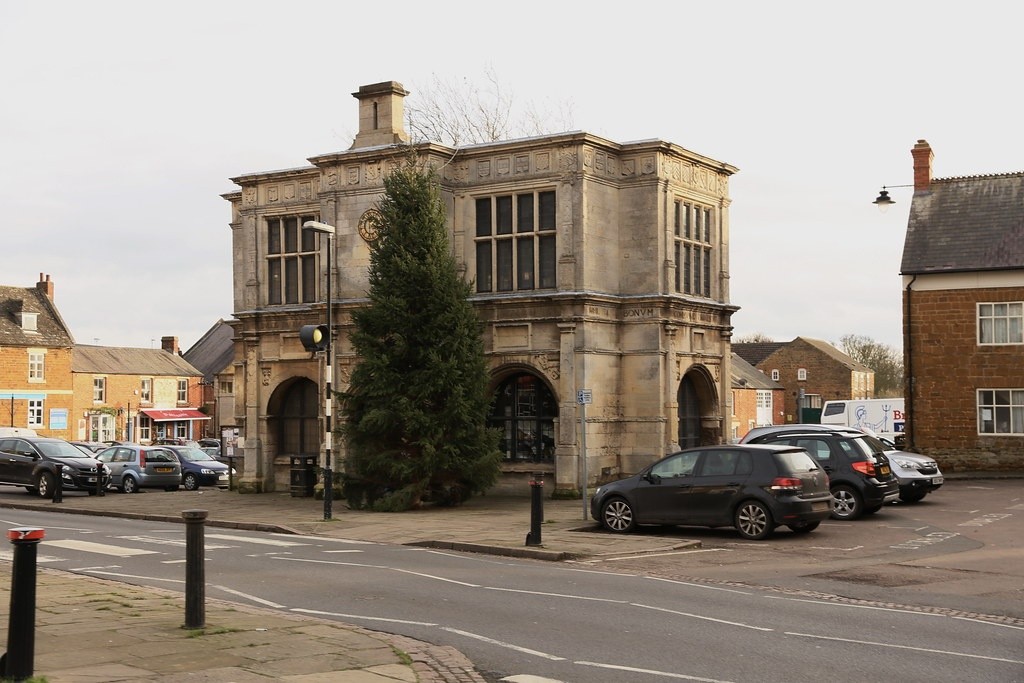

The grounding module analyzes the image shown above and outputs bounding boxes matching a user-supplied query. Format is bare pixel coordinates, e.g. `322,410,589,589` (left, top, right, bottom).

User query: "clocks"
358,208,383,241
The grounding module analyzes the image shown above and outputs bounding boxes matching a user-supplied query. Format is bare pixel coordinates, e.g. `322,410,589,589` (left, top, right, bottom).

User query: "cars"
0,436,113,499
70,438,222,458
158,445,237,491
93,445,183,494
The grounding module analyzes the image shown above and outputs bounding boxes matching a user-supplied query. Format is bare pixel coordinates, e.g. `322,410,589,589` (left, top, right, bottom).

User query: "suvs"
736,423,944,520
591,444,835,540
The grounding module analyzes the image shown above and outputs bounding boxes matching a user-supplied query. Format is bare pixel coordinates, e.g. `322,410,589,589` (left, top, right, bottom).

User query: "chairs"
702,453,722,475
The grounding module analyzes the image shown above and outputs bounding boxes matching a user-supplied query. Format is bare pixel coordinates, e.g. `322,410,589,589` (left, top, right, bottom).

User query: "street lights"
299,220,335,520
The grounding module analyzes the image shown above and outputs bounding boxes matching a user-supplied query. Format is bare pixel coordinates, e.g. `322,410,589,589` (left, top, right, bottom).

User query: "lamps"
873,184,915,213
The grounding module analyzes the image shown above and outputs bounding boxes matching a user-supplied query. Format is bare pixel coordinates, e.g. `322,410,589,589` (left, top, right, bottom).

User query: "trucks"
821,396,905,443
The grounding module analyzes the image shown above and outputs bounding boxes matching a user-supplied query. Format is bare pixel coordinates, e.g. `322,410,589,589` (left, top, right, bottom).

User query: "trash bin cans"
895,436,905,451
290,454,318,497
